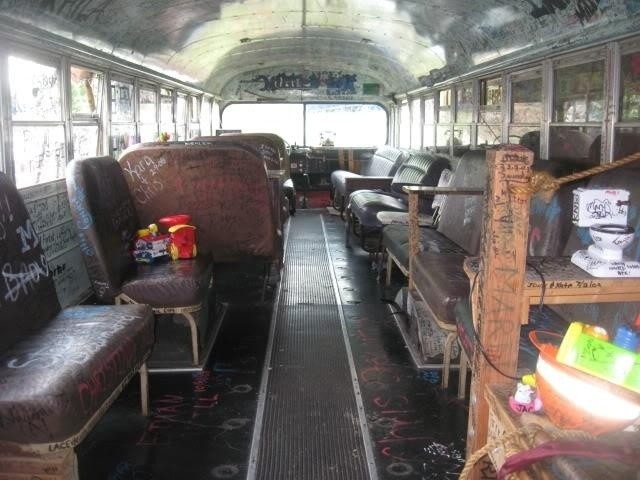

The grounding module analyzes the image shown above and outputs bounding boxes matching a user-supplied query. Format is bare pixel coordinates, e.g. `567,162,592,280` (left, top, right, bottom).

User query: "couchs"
117,142,285,301
342,153,454,252
66,155,216,365
453,165,639,400
1,173,157,476
331,145,402,212
380,150,601,390
